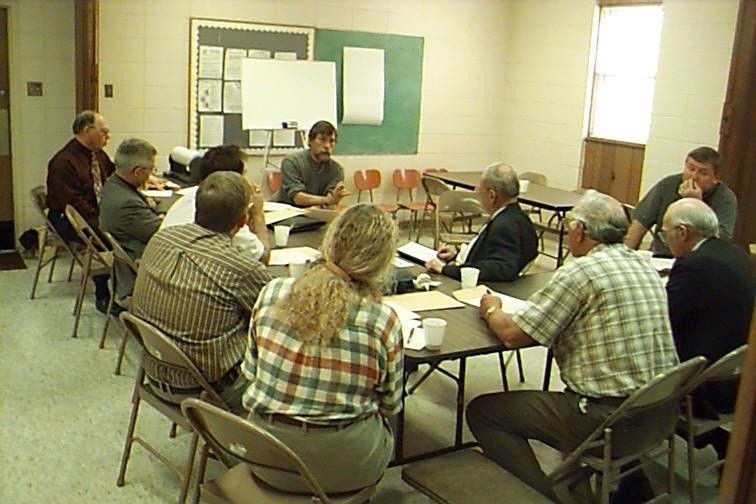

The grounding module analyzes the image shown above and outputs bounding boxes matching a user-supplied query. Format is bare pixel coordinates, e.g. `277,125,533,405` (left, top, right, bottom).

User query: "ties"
92,152,103,206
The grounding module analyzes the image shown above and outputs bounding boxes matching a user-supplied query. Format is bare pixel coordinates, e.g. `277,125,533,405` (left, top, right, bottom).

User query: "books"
397,241,448,271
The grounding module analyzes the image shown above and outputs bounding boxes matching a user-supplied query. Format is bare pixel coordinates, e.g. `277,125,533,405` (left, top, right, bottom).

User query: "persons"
464,189,681,504
656,198,756,487
131,172,273,415
623,146,737,256
97,137,164,302
394,163,539,373
45,111,128,317
241,203,404,494
156,144,271,267
265,120,351,207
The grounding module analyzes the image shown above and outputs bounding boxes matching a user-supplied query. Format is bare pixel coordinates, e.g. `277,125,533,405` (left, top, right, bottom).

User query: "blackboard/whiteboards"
240,57,338,131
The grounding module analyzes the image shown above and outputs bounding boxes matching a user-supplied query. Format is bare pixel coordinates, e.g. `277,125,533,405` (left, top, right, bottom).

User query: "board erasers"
283,122,298,130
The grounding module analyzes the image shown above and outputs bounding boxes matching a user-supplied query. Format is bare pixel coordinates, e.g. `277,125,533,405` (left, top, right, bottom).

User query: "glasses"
657,226,679,243
133,164,158,176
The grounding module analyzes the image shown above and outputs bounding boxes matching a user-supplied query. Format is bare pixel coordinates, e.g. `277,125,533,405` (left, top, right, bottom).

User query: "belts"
145,367,237,396
264,410,353,430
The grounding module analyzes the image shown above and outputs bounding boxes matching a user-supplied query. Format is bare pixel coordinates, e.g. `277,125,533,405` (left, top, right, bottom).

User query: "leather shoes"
97,295,128,316
609,476,655,504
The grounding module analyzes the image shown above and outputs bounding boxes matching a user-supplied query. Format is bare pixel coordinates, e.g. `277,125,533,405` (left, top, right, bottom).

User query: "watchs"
484,305,499,325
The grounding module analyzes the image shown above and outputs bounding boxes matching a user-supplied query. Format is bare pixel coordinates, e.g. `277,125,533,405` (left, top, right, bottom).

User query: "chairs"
622,204,636,224
65,204,113,337
181,397,384,504
518,171,546,251
673,344,748,504
99,226,138,375
30,185,84,298
117,312,250,504
269,171,283,193
354,168,492,250
547,355,707,504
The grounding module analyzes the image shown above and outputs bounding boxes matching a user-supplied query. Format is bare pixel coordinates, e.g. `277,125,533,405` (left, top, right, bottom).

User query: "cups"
519,179,530,194
274,225,290,247
637,250,653,264
423,317,447,351
460,266,481,289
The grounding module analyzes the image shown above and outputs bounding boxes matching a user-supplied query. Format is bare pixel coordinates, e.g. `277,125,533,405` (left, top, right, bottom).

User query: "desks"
423,172,581,271
146,175,553,468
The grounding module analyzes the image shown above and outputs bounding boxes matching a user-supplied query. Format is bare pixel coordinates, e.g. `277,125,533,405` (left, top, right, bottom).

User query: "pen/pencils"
407,328,415,343
